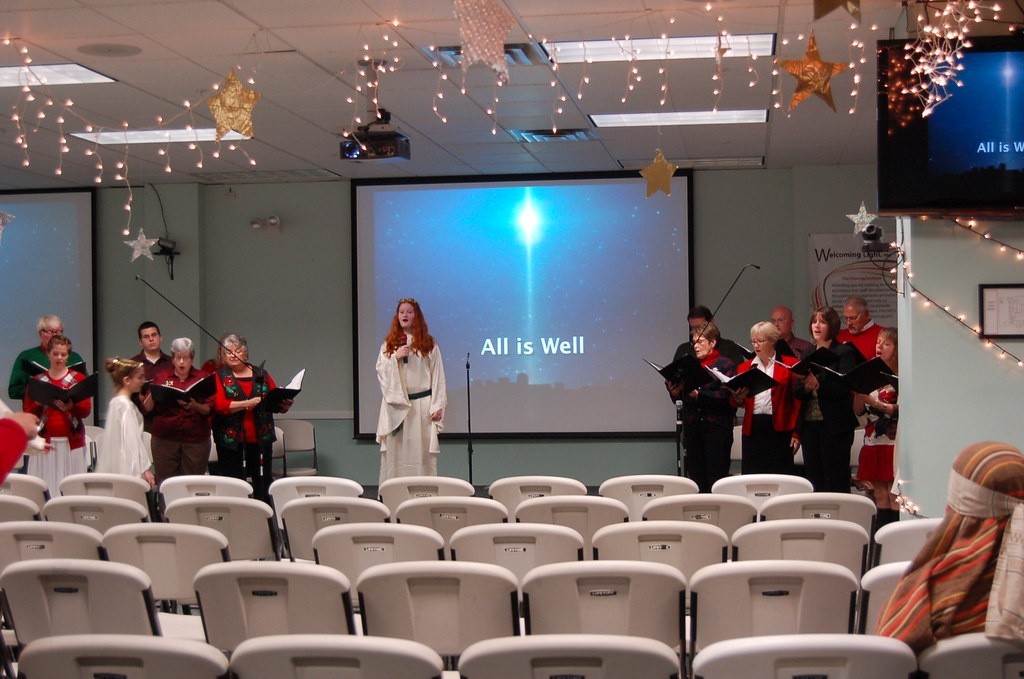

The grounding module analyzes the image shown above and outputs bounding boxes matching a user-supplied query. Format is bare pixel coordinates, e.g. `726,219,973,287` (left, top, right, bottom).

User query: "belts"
408,389,432,399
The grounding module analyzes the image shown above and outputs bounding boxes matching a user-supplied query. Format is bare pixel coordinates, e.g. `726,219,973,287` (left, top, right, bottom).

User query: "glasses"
691,339,709,345
844,313,863,322
751,339,768,345
42,329,63,336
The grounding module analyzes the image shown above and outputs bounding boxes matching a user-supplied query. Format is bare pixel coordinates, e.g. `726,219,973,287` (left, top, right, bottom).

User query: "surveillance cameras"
861,223,884,245
157,238,176,250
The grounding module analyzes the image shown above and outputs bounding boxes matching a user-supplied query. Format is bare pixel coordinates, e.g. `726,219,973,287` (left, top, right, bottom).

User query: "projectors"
339,139,411,164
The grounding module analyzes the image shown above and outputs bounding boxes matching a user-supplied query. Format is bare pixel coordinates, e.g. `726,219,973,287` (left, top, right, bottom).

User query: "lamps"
154,238,180,280
249,213,282,232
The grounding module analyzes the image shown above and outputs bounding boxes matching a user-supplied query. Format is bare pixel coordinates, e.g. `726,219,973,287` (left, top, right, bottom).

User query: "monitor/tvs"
874,35,1024,209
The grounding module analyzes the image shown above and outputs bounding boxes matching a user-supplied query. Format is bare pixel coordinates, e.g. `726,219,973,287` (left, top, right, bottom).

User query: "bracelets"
873,399,880,408
881,403,887,412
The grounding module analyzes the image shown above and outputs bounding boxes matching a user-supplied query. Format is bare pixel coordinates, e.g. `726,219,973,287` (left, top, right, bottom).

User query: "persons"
95,358,155,490
0,398,57,471
0,411,41,487
126,319,212,483
6,315,91,400
662,306,747,493
23,335,93,448
374,297,447,499
733,319,804,475
207,333,294,502
834,297,886,432
877,440,1024,679
852,325,900,532
769,305,813,360
796,306,861,495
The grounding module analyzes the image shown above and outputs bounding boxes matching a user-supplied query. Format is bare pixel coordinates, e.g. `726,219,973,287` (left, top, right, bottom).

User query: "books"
642,354,780,399
254,369,306,414
149,373,218,410
19,356,98,412
770,341,898,396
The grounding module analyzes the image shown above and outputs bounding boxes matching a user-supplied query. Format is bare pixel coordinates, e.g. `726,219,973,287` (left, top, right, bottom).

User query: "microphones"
690,263,760,354
134,275,259,375
400,334,409,363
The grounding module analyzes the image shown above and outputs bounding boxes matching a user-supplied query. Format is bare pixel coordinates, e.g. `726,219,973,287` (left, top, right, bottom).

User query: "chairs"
0,418,1024,679
